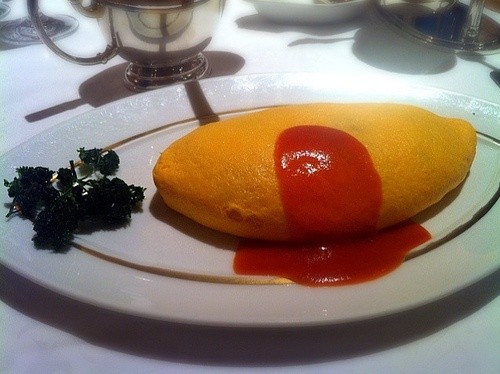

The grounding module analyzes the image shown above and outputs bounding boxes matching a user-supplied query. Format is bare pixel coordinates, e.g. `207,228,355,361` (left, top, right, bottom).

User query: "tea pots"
27,0,226,93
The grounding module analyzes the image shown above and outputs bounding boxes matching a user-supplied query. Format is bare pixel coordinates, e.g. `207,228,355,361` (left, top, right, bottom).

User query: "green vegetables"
5,147,148,252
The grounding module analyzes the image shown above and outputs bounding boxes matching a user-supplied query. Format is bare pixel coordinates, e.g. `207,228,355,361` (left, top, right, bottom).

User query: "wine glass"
0,0,79,47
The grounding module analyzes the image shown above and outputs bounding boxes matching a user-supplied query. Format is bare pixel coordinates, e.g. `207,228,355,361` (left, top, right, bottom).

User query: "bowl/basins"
244,0,374,24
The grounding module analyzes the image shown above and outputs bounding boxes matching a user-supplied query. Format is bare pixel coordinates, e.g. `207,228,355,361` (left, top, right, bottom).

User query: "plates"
1,72,498,329
